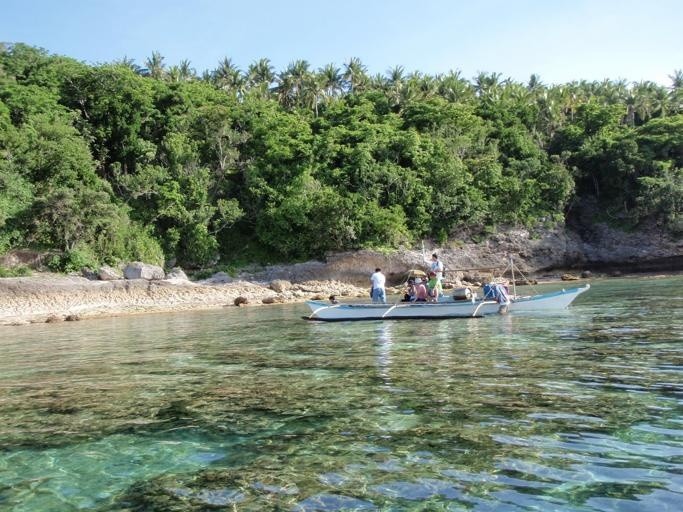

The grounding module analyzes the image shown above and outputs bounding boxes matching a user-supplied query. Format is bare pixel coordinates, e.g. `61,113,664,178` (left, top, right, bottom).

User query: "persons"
490,284,509,305
403,253,446,303
370,268,387,303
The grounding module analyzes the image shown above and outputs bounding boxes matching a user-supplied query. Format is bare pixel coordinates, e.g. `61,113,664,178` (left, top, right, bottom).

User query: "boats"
305,242,592,321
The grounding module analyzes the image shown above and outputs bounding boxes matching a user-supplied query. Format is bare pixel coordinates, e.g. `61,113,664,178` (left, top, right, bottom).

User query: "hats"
415,277,422,284
407,280,415,287
429,271,437,277
